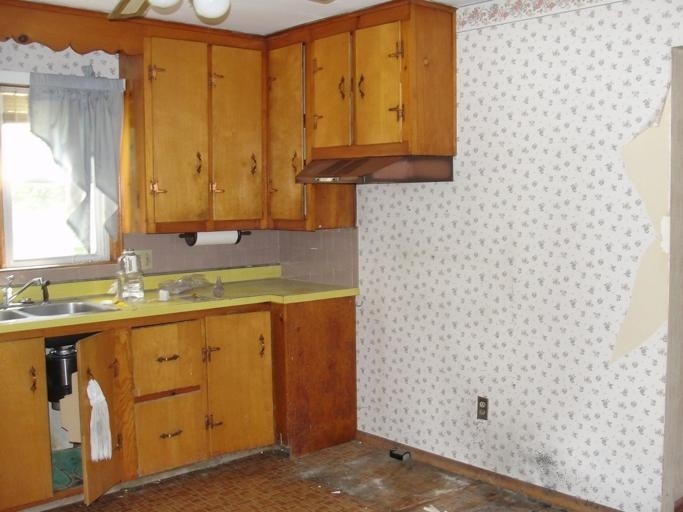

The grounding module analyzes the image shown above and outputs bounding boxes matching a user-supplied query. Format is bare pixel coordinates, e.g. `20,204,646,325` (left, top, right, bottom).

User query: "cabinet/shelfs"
305,0,458,161
125,302,277,487
0,319,124,511
117,17,357,237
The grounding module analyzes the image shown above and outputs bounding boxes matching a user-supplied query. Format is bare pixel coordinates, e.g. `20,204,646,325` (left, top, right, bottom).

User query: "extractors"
295,155,454,185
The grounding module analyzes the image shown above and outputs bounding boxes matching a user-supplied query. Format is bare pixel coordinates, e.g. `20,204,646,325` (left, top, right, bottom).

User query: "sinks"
17,301,101,316
0,307,26,321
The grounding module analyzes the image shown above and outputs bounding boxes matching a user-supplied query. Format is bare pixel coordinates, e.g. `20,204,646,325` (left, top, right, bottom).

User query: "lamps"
177,229,252,247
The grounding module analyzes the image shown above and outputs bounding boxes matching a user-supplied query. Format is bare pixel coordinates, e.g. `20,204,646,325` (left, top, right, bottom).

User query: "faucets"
5,274,43,306
